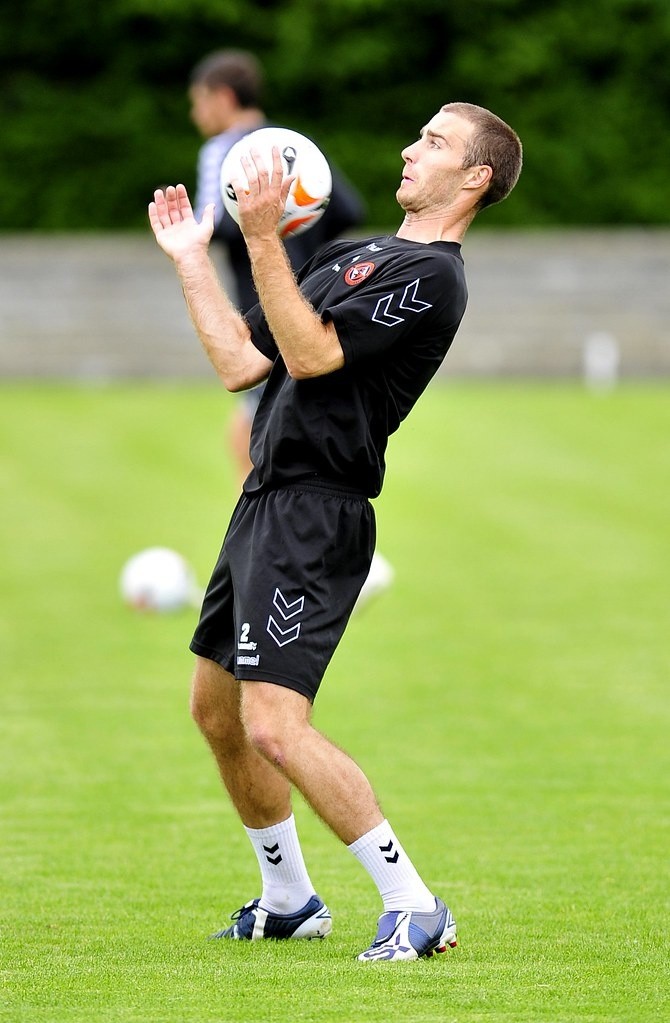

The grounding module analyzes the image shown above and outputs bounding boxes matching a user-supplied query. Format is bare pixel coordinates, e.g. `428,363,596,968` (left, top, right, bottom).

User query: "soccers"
219,126,332,242
118,546,196,616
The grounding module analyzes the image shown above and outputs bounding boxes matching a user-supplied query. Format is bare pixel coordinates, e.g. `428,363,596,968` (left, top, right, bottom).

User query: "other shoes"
352,554,391,603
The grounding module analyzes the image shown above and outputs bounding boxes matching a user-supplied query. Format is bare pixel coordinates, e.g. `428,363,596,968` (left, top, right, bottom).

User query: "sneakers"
356,893,458,964
209,893,334,942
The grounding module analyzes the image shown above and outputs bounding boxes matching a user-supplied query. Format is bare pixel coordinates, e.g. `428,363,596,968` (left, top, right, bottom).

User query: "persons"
149,103,522,962
192,49,367,479
